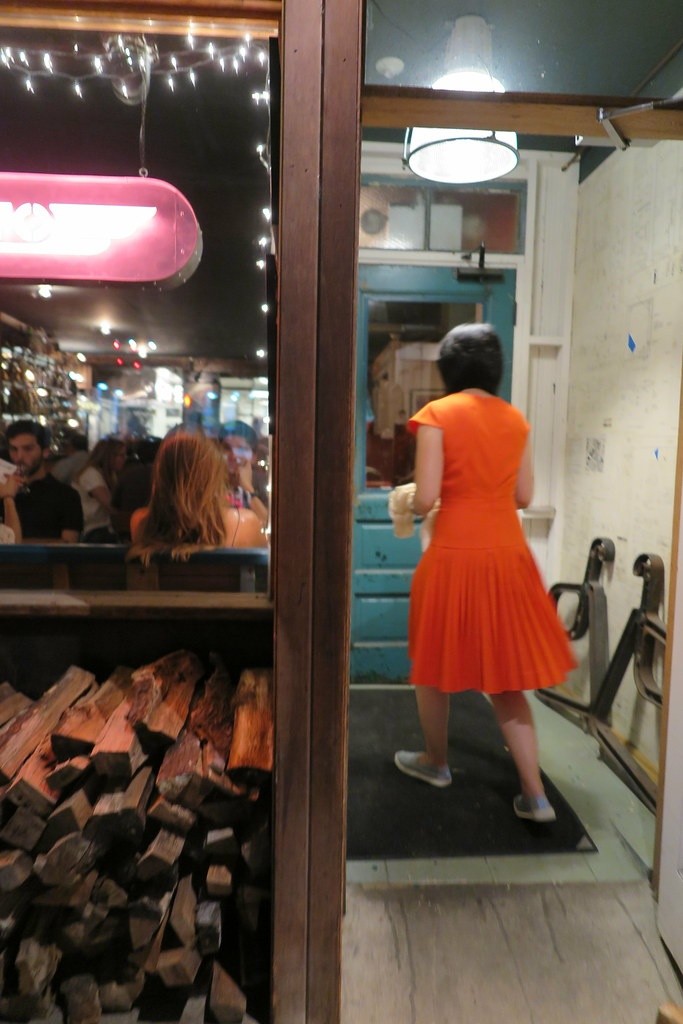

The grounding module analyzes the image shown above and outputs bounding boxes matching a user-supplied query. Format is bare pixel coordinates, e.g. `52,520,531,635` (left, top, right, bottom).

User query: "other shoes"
512,794,555,822
395,749,452,789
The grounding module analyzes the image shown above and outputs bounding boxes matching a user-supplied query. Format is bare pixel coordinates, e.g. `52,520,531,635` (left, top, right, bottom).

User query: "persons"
4,418,84,544
0,472,22,545
50,434,92,480
76,436,128,545
248,416,270,507
389,322,581,824
125,420,267,568
215,420,271,531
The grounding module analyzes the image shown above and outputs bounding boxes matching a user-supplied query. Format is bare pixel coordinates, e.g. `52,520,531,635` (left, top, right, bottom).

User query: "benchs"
0,540,271,705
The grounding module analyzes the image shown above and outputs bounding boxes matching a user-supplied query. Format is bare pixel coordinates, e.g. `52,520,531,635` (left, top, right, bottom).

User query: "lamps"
402,67,520,185
